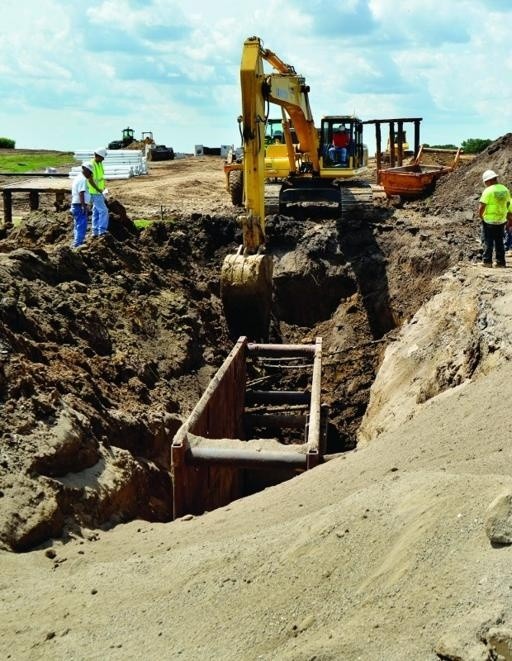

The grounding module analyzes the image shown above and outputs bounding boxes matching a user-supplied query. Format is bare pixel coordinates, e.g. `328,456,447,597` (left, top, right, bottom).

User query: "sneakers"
481,263,506,267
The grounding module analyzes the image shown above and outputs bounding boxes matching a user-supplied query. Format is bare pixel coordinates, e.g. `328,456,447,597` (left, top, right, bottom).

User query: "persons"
85,148,111,238
477,169,512,269
70,161,94,247
329,125,348,166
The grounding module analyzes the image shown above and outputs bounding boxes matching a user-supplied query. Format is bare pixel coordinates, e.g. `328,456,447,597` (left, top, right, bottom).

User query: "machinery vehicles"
108,127,175,161
375,130,413,162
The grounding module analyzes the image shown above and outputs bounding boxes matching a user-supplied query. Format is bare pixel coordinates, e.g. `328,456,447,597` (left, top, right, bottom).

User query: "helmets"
94,148,105,158
482,170,498,182
339,126,345,131
82,162,94,174
